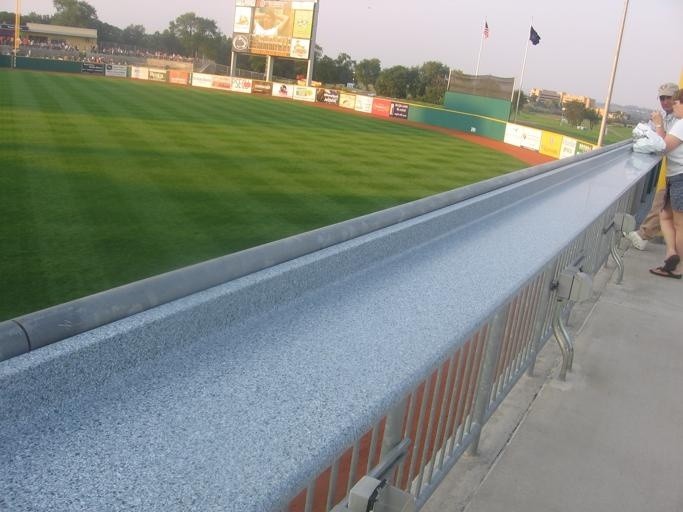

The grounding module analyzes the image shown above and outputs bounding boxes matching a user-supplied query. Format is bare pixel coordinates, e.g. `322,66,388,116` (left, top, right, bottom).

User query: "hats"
657,83,679,100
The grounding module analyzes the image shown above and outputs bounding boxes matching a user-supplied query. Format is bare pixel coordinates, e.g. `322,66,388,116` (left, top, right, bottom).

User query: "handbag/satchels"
632,123,666,153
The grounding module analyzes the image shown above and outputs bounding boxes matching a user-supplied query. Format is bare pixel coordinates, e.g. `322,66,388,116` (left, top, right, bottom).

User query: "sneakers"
623,230,649,251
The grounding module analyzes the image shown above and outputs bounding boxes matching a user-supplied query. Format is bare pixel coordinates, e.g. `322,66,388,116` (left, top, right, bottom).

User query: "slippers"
650,255,681,279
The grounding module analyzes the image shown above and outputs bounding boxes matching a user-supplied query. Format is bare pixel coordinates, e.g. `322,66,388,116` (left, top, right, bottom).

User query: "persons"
620,78,680,252
646,88,683,281
0,35,200,70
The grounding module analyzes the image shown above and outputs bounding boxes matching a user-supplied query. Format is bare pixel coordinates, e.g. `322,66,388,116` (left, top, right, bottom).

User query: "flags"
483,21,491,40
528,26,540,46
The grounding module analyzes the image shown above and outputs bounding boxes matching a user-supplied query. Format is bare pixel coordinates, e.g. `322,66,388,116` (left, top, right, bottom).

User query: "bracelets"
656,124,662,128
